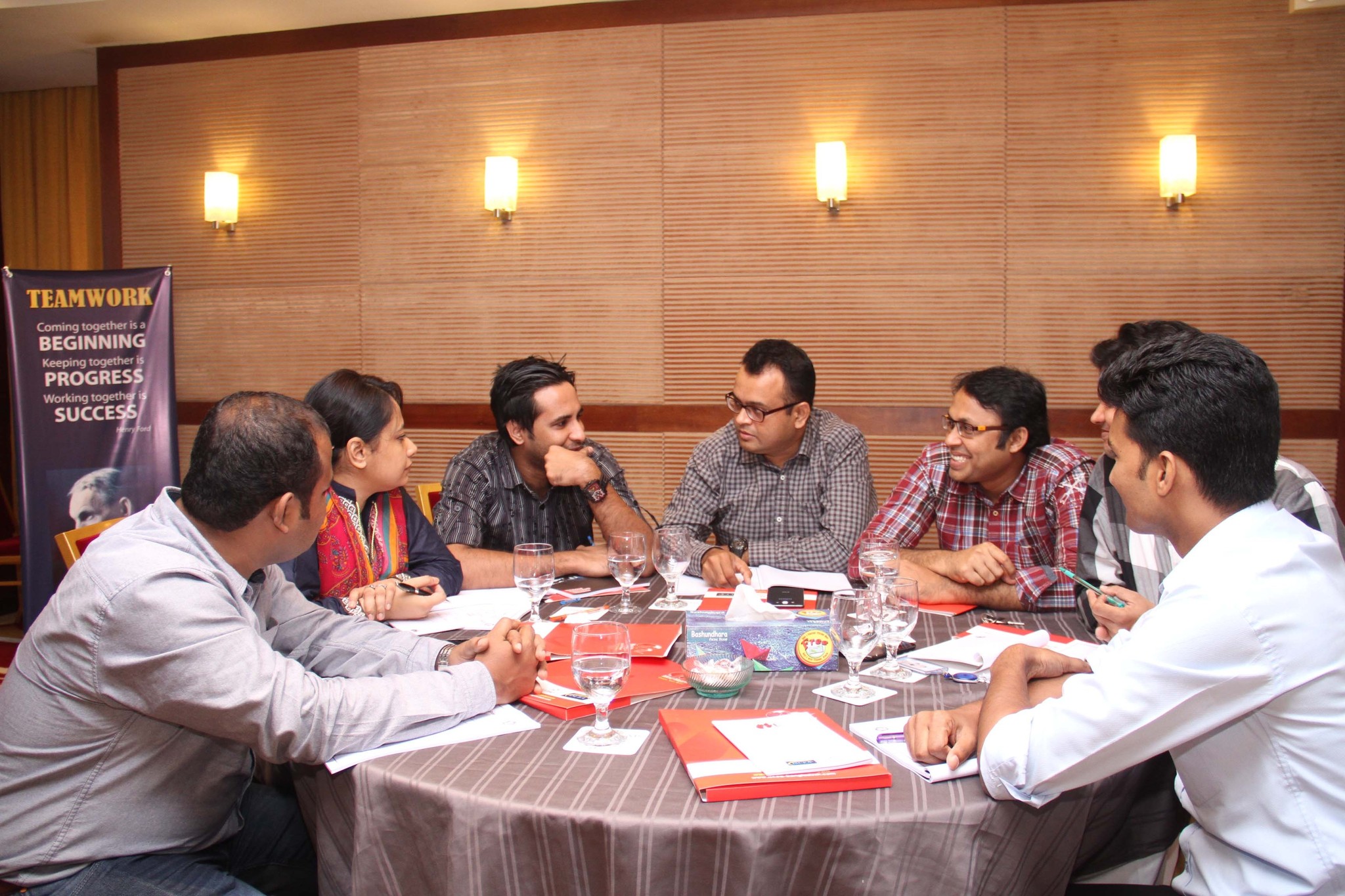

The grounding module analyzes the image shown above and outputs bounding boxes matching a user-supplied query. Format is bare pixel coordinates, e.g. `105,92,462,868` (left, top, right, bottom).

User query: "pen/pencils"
396,582,451,602
549,604,611,622
1057,565,1126,609
877,732,906,746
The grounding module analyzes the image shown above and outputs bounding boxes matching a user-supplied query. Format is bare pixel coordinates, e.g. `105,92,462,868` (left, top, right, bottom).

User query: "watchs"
727,537,749,559
580,468,609,503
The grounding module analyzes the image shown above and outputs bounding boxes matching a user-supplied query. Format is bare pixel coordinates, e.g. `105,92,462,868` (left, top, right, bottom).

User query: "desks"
291,566,1106,896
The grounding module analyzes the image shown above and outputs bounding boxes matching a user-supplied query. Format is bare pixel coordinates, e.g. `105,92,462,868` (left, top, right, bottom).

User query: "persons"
903,331,1344,896
65,467,138,531
845,364,1099,614
0,388,549,896
1071,316,1345,643
652,336,878,593
428,352,664,593
251,367,464,801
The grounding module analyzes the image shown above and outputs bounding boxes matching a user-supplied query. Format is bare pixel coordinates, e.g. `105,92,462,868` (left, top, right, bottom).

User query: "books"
847,716,980,784
711,710,873,777
320,702,542,775
547,573,651,600
446,586,546,631
674,564,856,596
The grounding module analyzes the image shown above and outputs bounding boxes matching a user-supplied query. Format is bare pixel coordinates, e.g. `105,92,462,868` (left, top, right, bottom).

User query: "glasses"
941,414,1008,439
725,392,800,423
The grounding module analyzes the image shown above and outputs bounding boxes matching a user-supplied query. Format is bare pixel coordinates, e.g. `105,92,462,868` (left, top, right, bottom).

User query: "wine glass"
513,542,557,625
829,587,884,702
607,531,647,615
866,576,920,681
651,527,692,611
857,537,899,621
570,620,631,746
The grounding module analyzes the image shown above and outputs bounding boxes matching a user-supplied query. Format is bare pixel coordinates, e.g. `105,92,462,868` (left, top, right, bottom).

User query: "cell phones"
768,586,804,609
446,638,472,646
863,641,915,661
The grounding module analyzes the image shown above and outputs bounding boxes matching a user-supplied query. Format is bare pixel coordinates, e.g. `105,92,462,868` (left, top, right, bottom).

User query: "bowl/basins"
680,652,753,699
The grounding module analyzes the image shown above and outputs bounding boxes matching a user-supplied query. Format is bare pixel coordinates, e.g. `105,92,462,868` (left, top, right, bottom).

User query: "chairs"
416,482,443,529
55,515,129,572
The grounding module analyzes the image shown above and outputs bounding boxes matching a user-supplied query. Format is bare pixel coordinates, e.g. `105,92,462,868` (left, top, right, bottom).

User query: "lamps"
816,140,847,212
204,170,240,233
1158,134,1196,207
484,155,518,222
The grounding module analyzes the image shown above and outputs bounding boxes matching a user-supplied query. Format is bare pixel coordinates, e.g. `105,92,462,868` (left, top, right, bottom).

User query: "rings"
365,584,375,590
376,584,387,589
